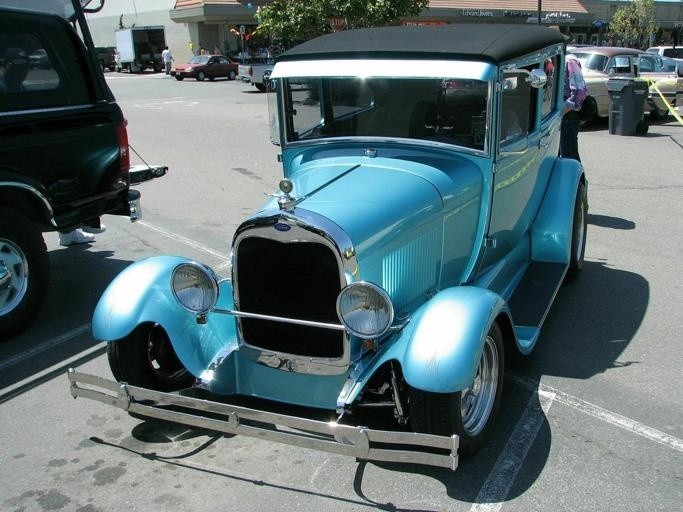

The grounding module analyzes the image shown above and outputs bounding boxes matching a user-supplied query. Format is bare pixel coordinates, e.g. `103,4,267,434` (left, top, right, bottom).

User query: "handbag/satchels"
563,95,594,120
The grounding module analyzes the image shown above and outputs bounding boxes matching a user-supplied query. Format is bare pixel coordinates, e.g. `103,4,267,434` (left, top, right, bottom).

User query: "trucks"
111,24,167,74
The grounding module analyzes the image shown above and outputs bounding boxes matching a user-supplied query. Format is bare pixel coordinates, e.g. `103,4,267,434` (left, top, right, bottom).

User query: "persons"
161,46,175,75
214,45,222,55
58,225,105,246
0,48,34,93
550,26,587,158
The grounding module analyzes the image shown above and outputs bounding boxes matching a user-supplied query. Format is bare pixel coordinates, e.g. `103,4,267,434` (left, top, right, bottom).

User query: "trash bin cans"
607,77,648,136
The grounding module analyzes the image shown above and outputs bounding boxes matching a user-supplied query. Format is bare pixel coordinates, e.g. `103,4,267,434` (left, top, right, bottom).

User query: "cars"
170,54,237,81
26,49,51,71
239,47,280,59
502,44,683,132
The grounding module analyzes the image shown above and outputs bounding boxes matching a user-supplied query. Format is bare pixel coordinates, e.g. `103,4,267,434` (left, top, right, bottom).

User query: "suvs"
67,24,588,473
86,46,116,73
1,0,169,343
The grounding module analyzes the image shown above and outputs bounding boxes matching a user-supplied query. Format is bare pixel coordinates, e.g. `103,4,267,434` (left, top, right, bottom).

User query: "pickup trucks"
237,64,310,91
